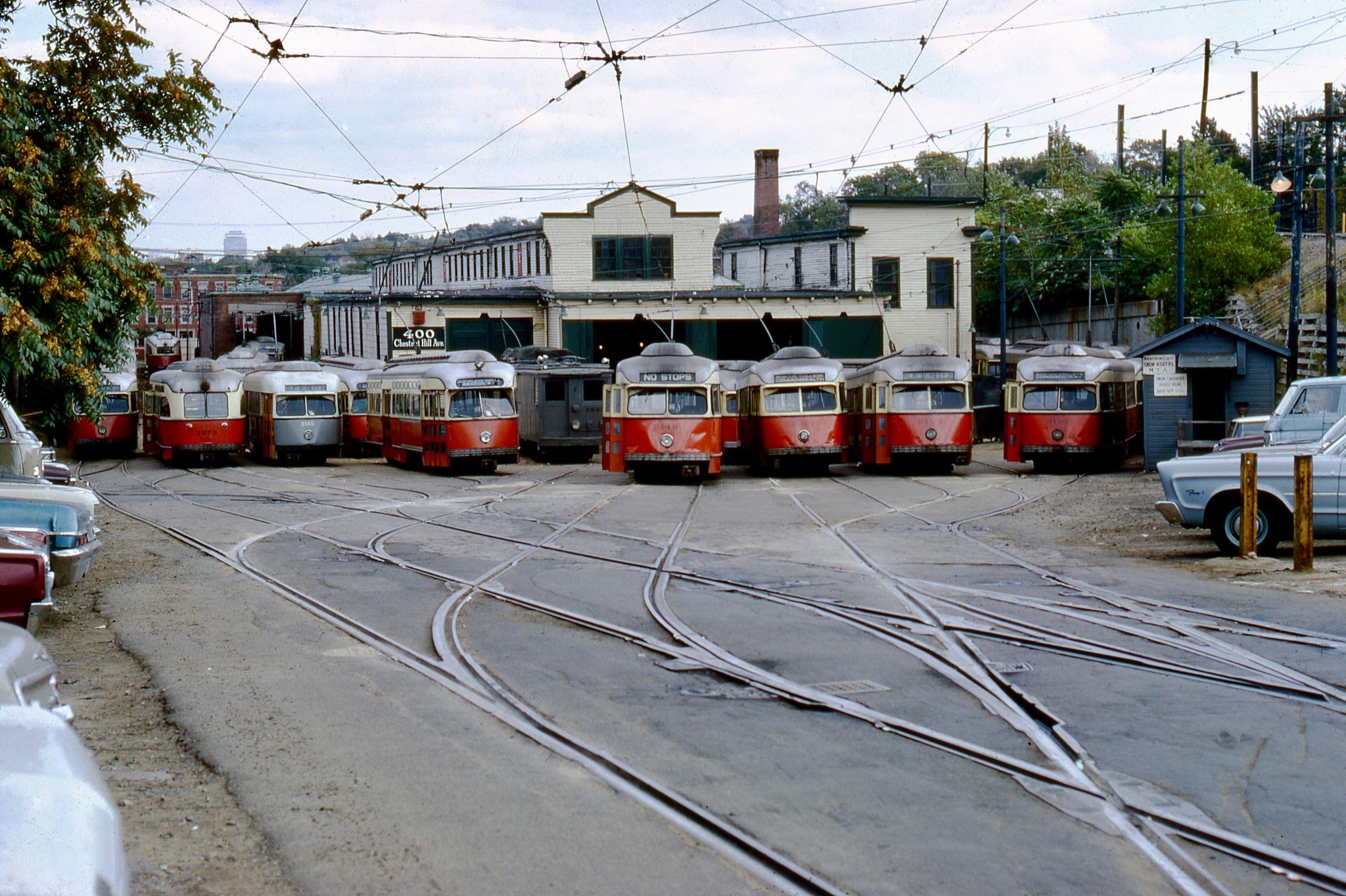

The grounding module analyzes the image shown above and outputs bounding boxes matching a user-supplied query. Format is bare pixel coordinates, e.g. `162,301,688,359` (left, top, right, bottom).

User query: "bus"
737,296,851,474
245,335,286,361
850,282,975,476
68,369,140,453
497,316,611,465
1001,260,1145,469
142,357,245,466
321,355,387,454
602,294,725,484
977,337,1042,380
145,332,183,371
367,309,520,478
244,312,341,466
217,345,271,374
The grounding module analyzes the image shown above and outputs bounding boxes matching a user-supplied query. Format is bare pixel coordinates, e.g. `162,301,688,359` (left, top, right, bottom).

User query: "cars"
1153,413,1346,557
0,398,105,638
1211,415,1271,452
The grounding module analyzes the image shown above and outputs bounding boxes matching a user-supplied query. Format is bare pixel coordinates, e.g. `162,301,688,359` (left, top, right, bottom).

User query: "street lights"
981,205,1020,434
1271,115,1346,371
1154,134,1207,332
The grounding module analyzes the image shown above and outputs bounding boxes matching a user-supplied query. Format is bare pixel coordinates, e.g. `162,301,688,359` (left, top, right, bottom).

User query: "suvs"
1263,375,1346,441
